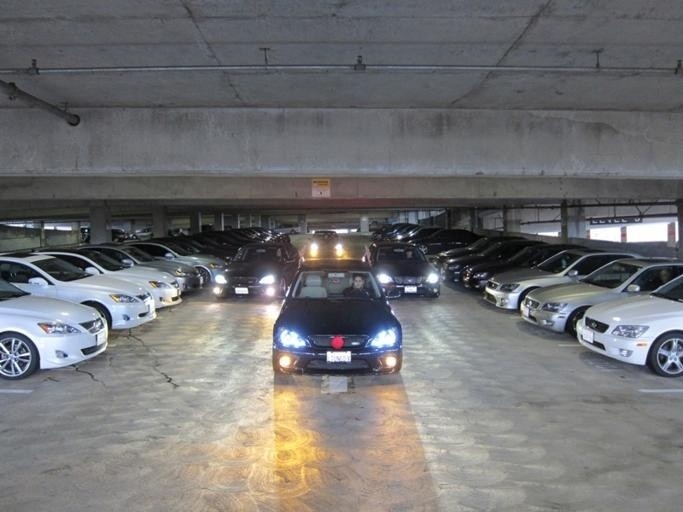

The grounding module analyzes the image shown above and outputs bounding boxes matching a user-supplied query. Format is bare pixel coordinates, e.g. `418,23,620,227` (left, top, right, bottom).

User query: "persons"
273,246,290,259
403,248,422,262
318,231,331,239
341,272,370,296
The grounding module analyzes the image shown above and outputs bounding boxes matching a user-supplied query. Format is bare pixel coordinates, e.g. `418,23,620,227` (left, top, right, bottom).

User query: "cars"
29,245,182,312
0,250,157,331
482,247,643,309
576,273,682,376
0,272,109,379
520,256,682,341
272,257,402,376
